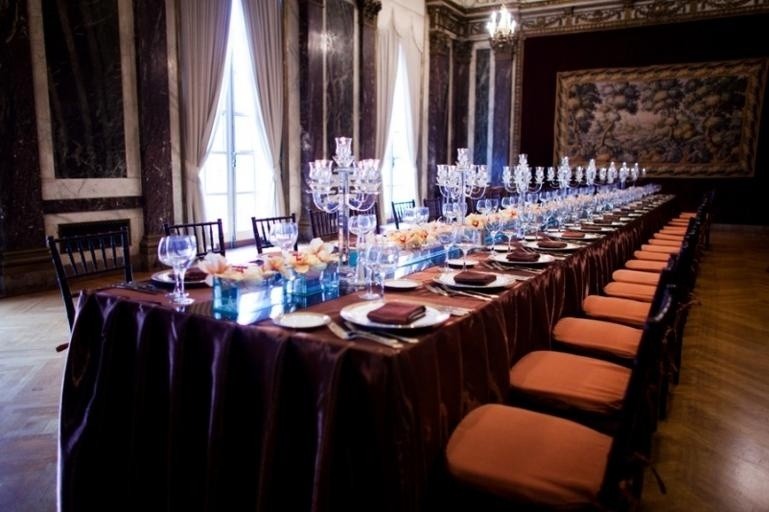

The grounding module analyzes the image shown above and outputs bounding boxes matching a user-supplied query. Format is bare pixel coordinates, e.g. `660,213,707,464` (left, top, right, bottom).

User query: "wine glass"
402,182,663,250
158,214,554,349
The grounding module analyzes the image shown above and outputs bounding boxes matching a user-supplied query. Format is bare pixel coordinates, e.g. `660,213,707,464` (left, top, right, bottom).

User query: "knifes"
112,281,166,298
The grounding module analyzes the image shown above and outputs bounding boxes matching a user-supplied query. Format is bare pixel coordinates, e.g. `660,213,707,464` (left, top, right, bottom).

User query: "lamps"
483,0,521,52
